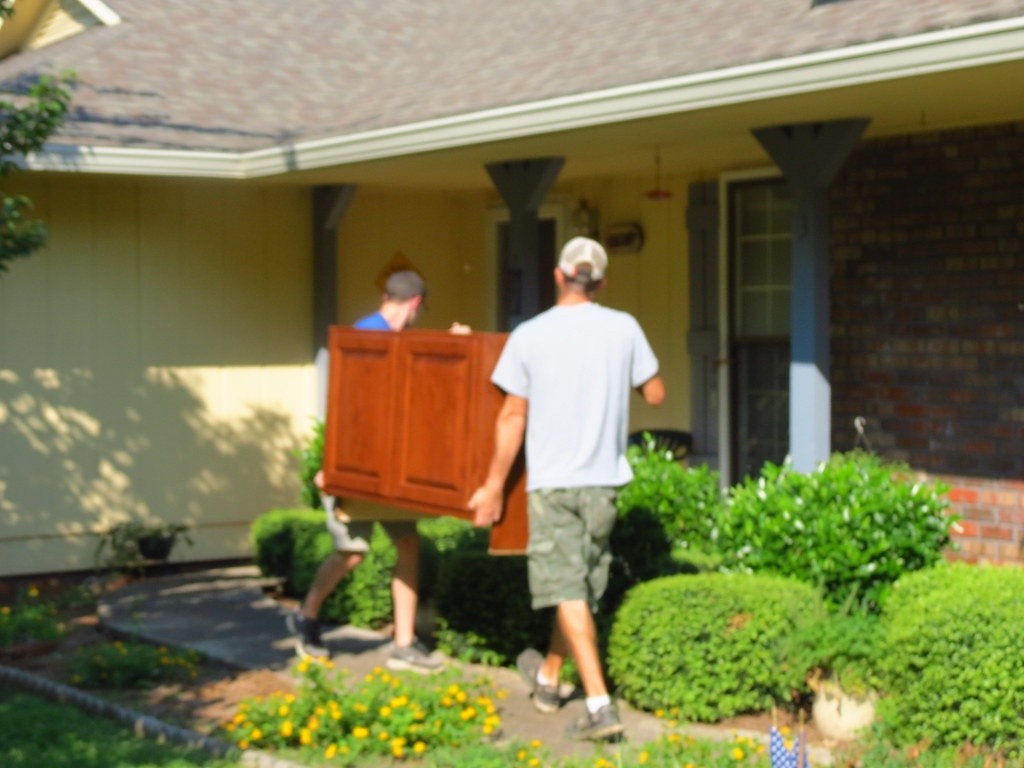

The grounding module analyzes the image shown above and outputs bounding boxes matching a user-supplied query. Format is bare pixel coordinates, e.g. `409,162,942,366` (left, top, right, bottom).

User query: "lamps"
642,155,672,202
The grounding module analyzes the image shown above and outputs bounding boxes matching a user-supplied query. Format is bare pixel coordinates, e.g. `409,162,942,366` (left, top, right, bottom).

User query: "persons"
468,237,666,740
283,271,471,676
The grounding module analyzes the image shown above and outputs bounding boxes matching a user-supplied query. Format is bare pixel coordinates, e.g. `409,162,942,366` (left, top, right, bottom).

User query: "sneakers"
518,647,561,711
567,704,621,740
286,608,332,659
388,639,445,674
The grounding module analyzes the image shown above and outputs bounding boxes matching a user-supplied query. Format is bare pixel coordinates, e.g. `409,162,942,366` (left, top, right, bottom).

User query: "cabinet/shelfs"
325,325,527,555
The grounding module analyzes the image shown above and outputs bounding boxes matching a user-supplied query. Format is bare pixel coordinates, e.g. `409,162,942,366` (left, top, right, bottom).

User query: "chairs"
627,428,693,464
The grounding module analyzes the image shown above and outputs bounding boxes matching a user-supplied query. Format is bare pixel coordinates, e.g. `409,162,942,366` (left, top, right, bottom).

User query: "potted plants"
94,520,194,581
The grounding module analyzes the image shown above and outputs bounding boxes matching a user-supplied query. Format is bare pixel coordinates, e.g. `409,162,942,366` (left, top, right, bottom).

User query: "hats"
560,235,608,283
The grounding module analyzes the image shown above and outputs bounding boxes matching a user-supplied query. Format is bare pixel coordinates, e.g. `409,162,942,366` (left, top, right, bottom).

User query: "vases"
810,680,878,739
